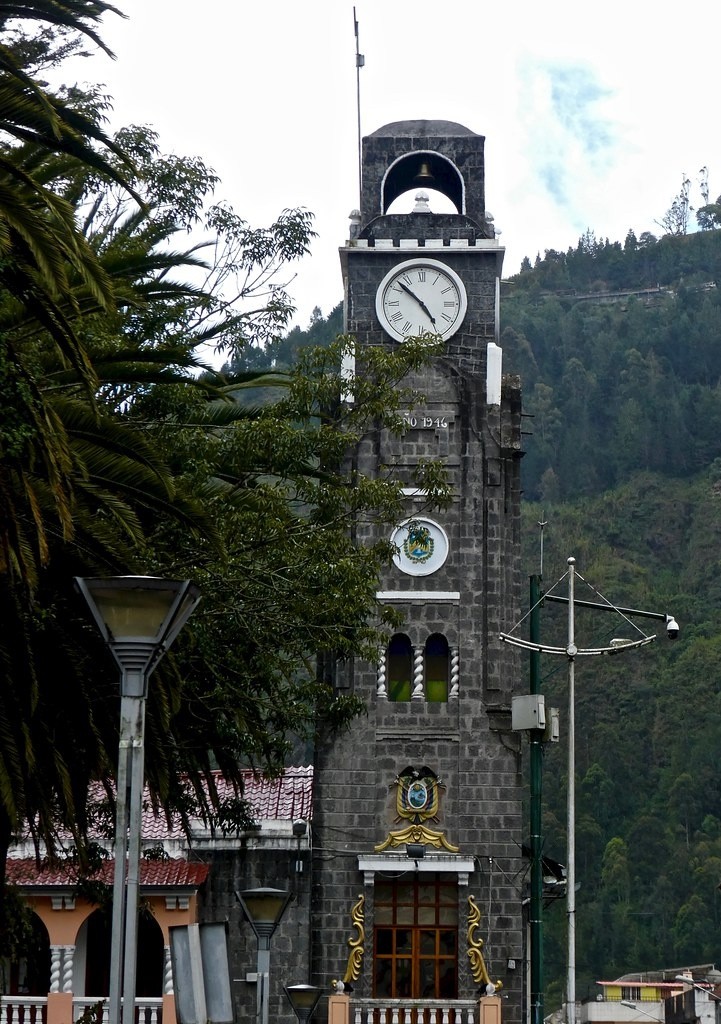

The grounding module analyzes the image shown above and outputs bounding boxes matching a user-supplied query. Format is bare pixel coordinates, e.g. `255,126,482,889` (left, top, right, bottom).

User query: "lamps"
294,818,307,874
405,842,427,858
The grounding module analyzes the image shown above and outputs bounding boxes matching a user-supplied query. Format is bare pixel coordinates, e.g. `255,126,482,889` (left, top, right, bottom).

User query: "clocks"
374,256,468,348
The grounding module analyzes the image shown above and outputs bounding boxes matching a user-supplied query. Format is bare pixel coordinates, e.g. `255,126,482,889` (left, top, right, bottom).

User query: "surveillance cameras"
667,621,680,641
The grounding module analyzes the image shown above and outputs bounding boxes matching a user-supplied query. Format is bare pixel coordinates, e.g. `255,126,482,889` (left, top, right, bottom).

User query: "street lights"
500,555,681,1024
283,985,329,1024
235,888,300,1024
73,573,205,1023
